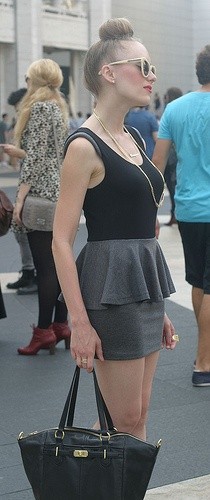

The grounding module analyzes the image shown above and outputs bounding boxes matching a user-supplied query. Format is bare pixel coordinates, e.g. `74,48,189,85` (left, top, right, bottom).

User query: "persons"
51,18,179,440
0,87,37,294
12,59,71,355
152,46,210,386
127,87,183,226
69,112,90,133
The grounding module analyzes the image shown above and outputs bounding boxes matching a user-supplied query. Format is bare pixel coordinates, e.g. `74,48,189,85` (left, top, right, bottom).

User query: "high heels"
18,324,57,356
40,320,71,349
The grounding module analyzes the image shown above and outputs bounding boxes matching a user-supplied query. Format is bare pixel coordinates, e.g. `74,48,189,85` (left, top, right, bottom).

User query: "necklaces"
93,111,165,207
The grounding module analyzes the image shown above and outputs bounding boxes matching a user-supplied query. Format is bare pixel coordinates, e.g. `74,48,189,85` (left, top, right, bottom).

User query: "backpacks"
0,191,14,237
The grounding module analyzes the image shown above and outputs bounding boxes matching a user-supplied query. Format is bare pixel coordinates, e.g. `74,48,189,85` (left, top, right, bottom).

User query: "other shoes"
7,275,34,289
17,280,38,295
191,370,210,387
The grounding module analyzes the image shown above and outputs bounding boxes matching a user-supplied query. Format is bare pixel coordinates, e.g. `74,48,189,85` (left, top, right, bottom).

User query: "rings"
81,359,87,363
172,335,179,341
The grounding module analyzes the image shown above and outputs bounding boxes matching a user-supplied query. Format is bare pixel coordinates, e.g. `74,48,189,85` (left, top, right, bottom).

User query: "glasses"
98,56,157,78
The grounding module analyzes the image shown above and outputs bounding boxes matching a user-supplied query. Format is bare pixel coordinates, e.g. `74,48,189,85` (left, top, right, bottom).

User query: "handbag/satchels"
19,195,58,232
18,363,163,500
